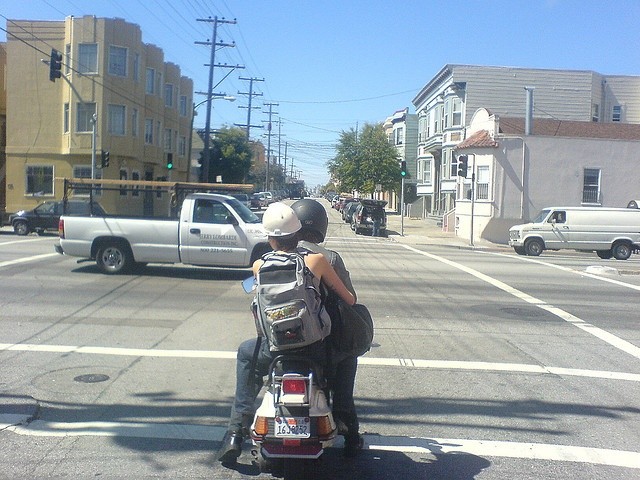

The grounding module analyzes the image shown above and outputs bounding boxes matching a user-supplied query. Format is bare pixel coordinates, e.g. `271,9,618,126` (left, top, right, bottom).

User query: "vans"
507,205,640,261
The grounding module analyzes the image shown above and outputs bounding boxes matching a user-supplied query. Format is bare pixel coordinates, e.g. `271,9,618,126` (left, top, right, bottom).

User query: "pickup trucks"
52,189,271,275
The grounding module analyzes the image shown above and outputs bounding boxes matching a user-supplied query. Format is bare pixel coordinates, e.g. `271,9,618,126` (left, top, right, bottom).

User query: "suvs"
352,199,389,235
8,199,105,236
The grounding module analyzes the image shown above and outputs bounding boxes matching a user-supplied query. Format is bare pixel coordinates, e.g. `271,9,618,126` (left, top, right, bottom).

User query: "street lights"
185,95,237,183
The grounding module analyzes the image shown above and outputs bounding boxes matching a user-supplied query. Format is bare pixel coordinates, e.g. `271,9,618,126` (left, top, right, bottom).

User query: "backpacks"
250,246,340,386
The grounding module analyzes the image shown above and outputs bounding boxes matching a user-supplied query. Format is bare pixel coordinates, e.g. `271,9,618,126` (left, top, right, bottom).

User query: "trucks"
288,179,305,199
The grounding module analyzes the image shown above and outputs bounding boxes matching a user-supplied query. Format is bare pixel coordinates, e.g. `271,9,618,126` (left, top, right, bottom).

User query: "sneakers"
333,411,348,435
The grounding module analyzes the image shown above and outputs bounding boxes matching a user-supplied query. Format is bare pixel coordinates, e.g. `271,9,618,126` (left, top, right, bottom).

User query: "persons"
371,206,380,237
233,202,358,437
216,198,364,465
552,213,559,223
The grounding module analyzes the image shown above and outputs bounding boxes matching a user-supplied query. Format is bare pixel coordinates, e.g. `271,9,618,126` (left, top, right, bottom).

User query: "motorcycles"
240,272,344,478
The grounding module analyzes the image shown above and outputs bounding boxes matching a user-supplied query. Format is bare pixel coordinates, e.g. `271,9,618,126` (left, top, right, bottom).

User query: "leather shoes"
344,437,364,458
218,428,243,463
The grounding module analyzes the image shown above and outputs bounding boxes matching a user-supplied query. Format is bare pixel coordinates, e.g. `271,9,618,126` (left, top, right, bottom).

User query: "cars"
235,189,285,209
323,192,359,221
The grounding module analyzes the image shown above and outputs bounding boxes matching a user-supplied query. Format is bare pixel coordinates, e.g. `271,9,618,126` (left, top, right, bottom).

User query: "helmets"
292,199,328,243
260,202,302,237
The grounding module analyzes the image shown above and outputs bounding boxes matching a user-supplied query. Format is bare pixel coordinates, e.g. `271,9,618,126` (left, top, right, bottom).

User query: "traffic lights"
49,50,62,81
101,151,110,168
458,155,466,177
401,161,406,177
166,152,173,170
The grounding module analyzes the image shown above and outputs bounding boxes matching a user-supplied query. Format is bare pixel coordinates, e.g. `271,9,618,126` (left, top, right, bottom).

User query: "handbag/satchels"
324,294,372,357
